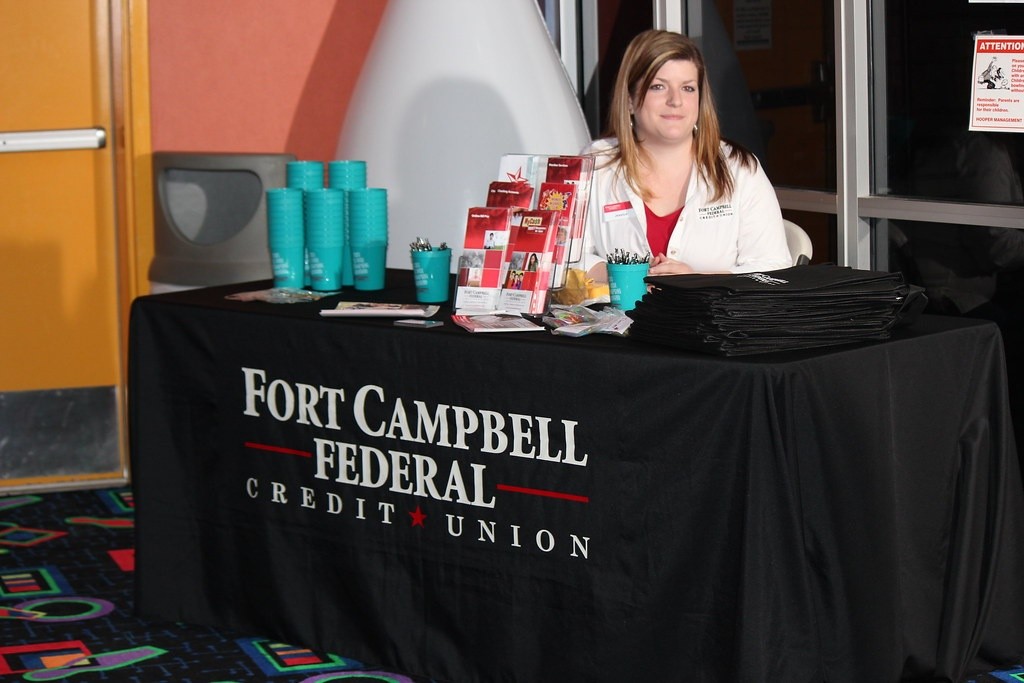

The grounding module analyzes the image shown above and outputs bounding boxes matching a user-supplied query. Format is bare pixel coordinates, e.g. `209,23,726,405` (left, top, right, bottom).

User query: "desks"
129,280,1024,683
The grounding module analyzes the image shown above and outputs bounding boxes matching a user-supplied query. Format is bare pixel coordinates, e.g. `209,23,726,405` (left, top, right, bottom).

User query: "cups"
410,246,450,303
266,162,388,291
607,263,650,311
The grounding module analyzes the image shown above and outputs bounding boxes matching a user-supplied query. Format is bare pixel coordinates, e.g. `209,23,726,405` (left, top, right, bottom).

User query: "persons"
484,232,495,248
528,252,538,273
565,29,793,293
508,270,524,289
